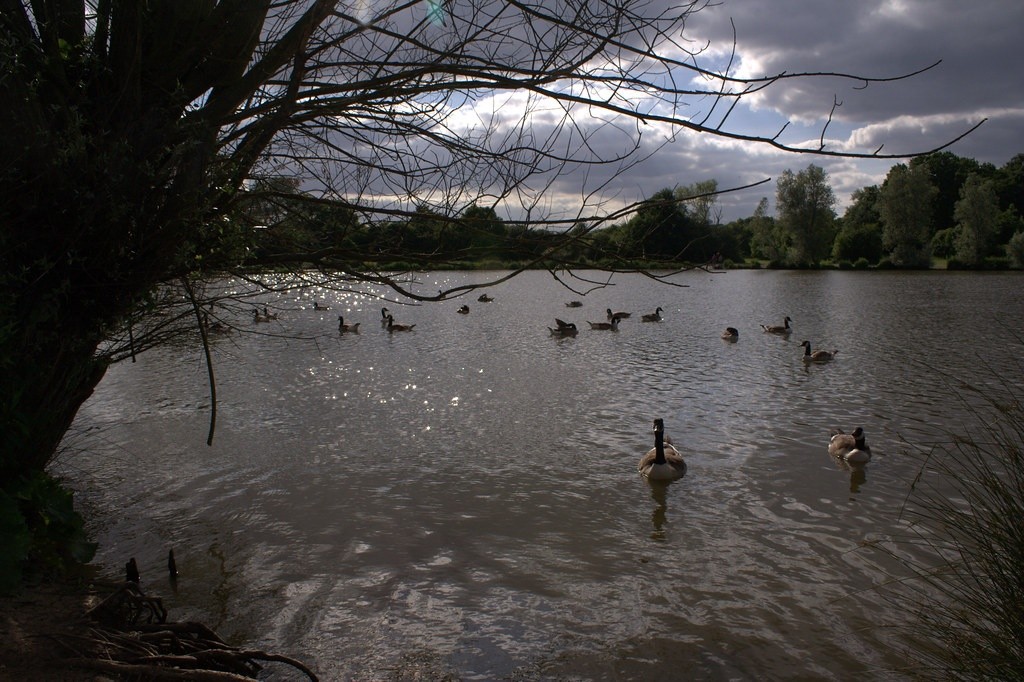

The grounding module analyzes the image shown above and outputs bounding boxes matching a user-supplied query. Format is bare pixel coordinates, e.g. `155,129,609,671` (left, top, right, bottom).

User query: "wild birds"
547,300,664,338
827,427,872,463
250,290,495,335
719,327,738,343
796,340,839,361
759,317,794,334
637,417,687,482
